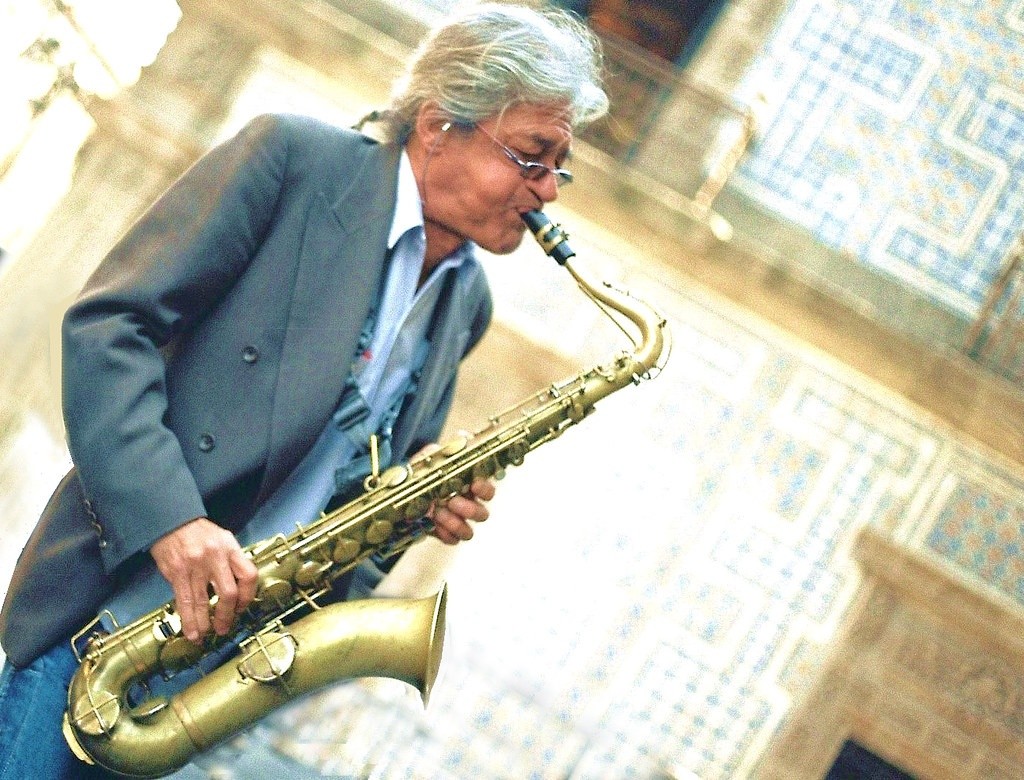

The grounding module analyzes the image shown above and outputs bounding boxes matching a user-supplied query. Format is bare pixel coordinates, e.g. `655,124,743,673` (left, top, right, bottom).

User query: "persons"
0,0,614,780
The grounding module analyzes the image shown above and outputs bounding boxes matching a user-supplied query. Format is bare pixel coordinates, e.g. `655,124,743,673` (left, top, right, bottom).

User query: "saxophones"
61,207,673,780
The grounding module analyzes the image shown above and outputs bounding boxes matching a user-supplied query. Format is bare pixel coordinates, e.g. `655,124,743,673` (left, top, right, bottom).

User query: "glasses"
472,120,575,189
